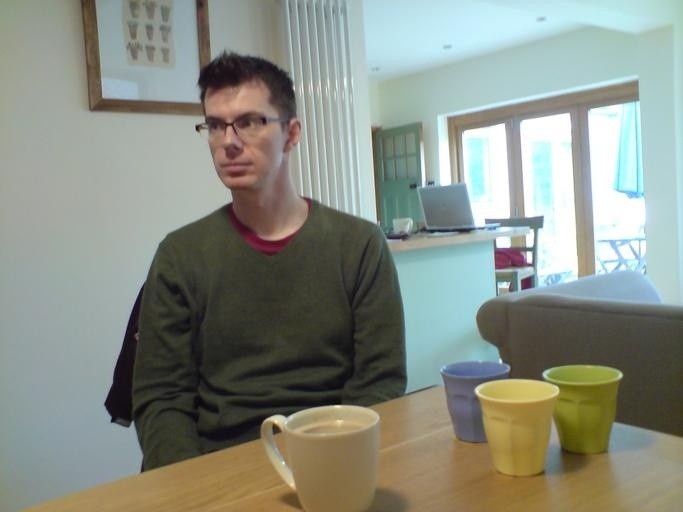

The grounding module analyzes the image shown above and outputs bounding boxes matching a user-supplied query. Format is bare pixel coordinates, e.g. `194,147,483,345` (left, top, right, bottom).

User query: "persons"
127,50,409,475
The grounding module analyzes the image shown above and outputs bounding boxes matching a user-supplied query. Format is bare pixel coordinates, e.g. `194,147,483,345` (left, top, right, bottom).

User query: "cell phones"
386,232,408,239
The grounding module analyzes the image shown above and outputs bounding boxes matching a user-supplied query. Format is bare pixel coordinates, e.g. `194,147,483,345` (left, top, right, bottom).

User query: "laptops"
417,182,501,232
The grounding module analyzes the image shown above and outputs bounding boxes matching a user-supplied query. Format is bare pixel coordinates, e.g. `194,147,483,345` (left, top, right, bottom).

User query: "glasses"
192,110,285,141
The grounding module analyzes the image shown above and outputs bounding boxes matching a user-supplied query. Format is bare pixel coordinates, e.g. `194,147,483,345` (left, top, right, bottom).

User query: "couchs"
473,268,683,437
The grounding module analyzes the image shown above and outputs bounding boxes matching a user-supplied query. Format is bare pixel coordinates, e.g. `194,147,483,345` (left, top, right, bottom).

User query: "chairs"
483,215,543,292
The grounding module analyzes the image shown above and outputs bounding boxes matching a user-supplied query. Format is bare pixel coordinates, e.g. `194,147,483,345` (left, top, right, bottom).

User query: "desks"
595,237,647,275
15,384,681,512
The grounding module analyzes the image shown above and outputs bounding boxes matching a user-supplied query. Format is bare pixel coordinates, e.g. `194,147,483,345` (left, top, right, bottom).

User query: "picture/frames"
80,1,213,117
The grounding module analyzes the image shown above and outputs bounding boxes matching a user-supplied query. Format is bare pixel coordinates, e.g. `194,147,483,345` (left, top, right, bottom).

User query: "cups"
392,218,412,233
475,378,561,478
542,364,623,455
259,404,382,511
439,359,512,443
